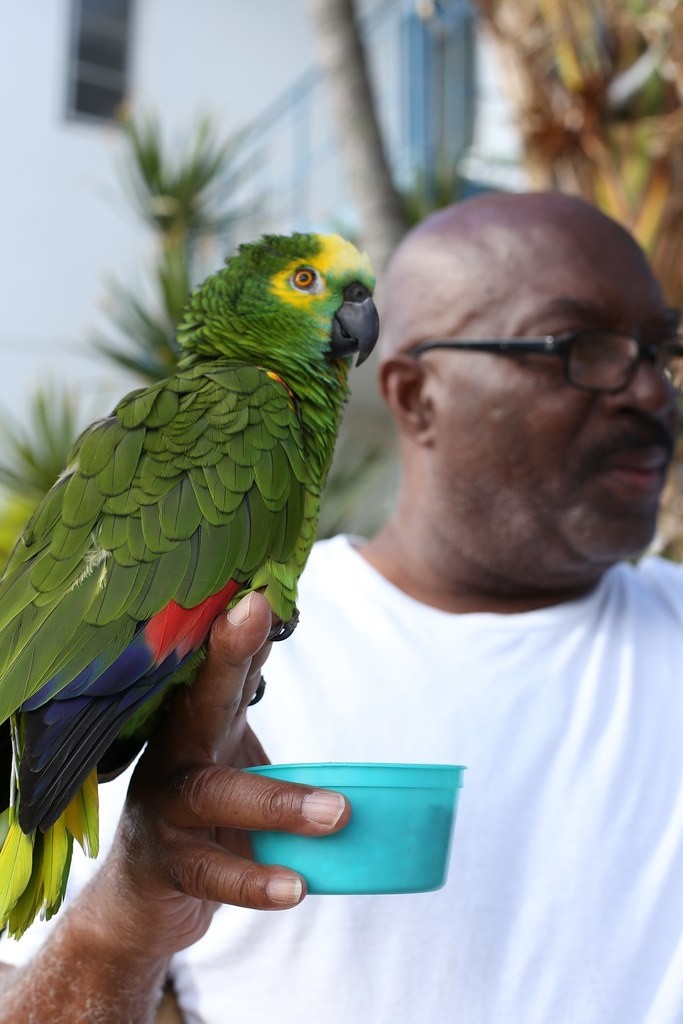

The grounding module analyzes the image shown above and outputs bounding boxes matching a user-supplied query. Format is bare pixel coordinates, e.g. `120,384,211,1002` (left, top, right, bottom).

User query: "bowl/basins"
241,763,467,895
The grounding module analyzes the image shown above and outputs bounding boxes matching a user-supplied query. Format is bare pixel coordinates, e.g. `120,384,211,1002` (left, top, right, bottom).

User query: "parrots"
1,230,380,941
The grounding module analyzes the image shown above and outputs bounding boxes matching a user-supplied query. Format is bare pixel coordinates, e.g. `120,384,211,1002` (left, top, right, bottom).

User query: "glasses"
407,328,683,399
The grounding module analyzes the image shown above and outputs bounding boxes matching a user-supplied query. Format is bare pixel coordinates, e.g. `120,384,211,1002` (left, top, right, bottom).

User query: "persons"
0,188,683,1024
